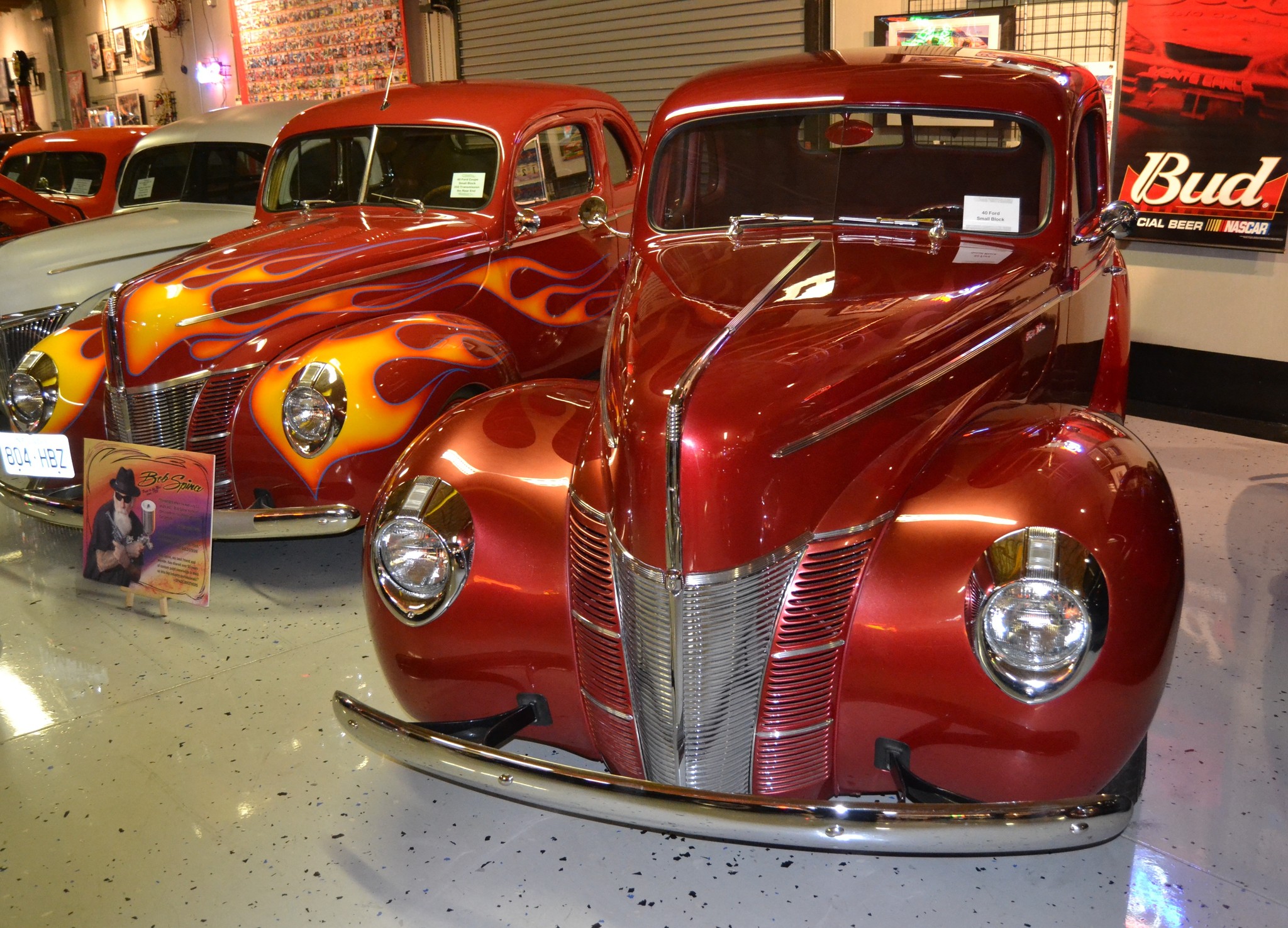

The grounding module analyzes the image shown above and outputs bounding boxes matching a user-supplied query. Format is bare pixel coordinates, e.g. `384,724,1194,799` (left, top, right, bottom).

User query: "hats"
109,466,141,497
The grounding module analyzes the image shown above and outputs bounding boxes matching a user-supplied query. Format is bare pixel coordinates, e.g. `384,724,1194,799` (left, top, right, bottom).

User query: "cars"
0,101,383,407
0,125,166,244
339,46,1190,858
1,81,644,542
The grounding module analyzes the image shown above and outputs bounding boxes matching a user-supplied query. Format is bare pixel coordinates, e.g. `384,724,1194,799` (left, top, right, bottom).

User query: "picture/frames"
112,26,127,55
86,105,110,128
86,32,103,79
103,48,117,71
114,90,143,126
132,24,157,73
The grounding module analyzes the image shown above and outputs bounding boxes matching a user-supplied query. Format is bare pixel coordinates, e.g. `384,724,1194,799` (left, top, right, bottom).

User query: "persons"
83,466,149,587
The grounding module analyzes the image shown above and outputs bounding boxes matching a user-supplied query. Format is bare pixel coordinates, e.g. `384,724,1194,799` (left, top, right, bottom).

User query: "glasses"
115,490,132,504
